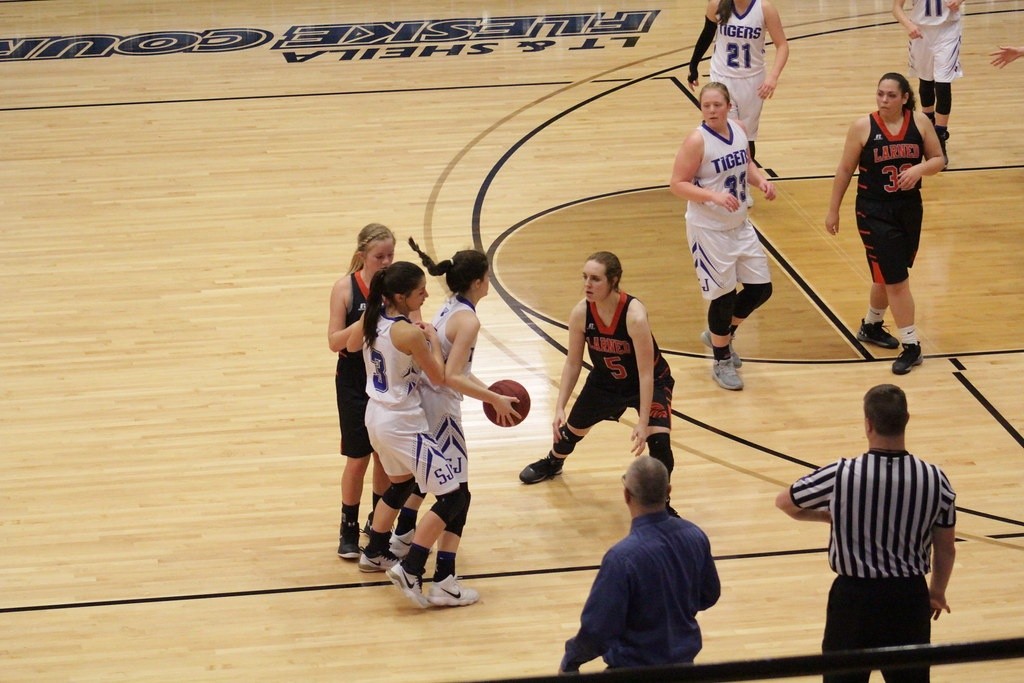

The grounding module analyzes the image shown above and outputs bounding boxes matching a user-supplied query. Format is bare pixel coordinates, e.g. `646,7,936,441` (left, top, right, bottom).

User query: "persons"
687,0,789,208
825,73,945,375
328,223,521,608
989,46,1024,68
671,82,776,390
775,384,956,683
892,0,966,169
557,456,721,674
519,251,681,520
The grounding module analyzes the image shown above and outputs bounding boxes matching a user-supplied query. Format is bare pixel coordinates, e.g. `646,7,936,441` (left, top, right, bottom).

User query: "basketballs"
482,380,531,427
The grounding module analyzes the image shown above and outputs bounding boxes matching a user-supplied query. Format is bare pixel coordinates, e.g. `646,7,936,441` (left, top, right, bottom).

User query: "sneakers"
938,133,949,168
700,325,743,369
712,358,743,390
364,511,376,535
358,543,403,573
388,529,414,557
338,524,362,559
892,340,923,375
428,569,480,606
519,455,564,484
666,499,681,519
857,317,900,348
386,558,428,609
